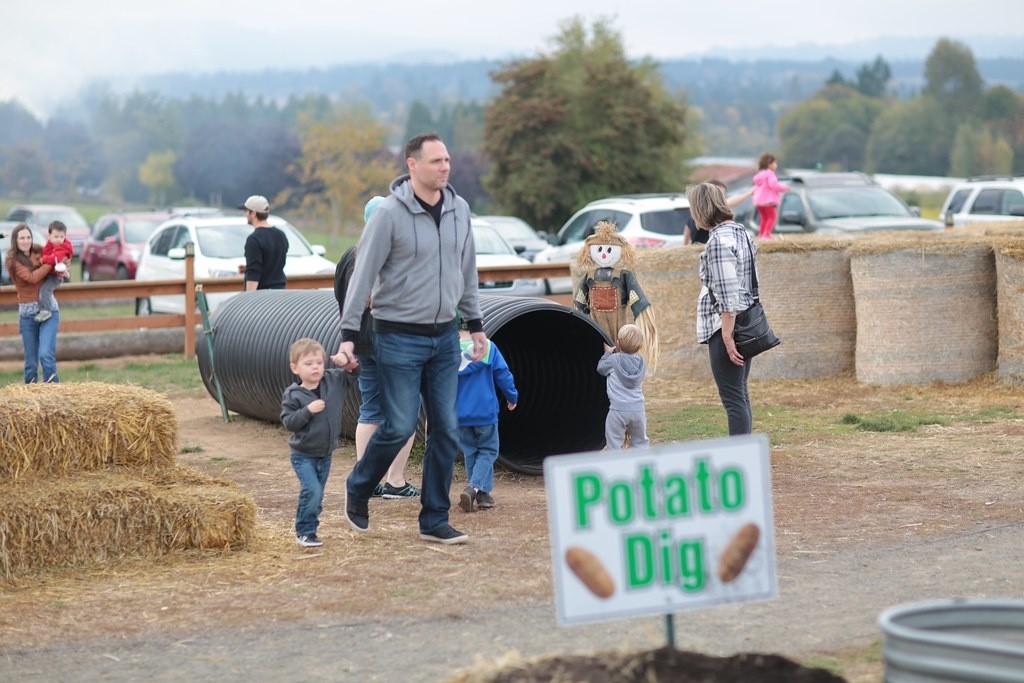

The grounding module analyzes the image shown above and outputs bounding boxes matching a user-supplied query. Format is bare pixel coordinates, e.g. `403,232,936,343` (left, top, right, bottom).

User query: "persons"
751,152,792,238
237,195,290,291
332,196,422,499
5,220,75,383
280,338,362,546
337,133,489,544
683,178,754,245
455,308,518,513
570,219,660,446
686,182,761,436
596,323,651,452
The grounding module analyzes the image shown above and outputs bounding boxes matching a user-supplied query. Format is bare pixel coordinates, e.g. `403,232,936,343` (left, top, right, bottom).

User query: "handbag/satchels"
732,302,782,361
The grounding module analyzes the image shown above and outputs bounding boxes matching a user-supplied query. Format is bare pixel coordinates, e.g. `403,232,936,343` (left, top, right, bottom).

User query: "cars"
81,208,223,282
469,212,547,298
0,205,91,284
133,217,337,317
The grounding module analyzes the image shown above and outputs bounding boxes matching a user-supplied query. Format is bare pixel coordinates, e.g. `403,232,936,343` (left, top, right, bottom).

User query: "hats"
363,195,386,222
238,194,270,215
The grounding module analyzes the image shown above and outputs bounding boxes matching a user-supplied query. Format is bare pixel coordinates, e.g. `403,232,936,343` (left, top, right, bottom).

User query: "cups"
54,262,66,279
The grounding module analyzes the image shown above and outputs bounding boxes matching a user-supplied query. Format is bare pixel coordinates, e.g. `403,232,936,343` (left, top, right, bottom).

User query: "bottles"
458,342,483,372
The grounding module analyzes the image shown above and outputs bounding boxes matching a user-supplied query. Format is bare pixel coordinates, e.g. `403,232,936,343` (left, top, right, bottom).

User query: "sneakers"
344,478,371,532
460,487,476,512
382,481,422,499
477,493,494,509
420,522,468,546
33,310,52,322
373,484,386,498
297,528,324,548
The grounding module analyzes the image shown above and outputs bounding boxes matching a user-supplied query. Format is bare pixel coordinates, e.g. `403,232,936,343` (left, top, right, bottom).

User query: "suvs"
725,168,1024,235
534,193,691,296
